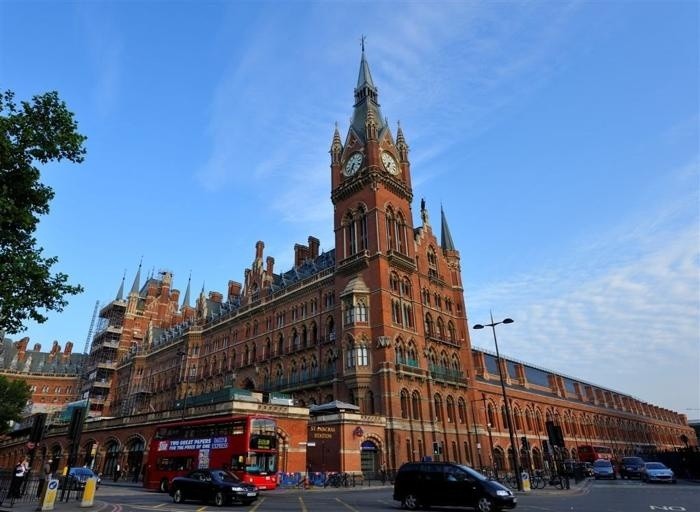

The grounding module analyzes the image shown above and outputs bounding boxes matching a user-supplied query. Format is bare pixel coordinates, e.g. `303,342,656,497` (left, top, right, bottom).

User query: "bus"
141,413,280,491
577,443,613,462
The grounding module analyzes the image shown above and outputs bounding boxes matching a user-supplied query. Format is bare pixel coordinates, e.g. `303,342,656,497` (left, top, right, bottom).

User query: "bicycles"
479,465,545,489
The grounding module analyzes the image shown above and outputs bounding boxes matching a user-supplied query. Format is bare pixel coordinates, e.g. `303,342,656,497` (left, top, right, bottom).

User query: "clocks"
381,150,401,176
341,150,364,178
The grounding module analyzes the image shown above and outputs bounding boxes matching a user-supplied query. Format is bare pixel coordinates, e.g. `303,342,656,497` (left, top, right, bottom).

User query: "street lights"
473,310,514,490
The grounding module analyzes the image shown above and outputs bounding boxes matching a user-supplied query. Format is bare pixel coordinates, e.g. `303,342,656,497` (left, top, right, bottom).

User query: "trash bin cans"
520,472,531,492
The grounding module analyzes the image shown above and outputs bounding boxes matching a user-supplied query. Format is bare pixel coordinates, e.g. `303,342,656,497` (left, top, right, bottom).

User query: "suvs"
618,456,644,479
391,462,517,511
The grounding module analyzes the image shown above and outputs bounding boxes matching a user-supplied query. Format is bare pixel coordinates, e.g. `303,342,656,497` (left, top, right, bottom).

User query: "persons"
35,459,54,499
80,460,140,485
6,458,23,505
21,455,32,495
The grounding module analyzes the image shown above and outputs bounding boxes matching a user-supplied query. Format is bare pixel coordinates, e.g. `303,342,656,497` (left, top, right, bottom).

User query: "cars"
55,467,101,491
168,468,260,507
639,462,677,484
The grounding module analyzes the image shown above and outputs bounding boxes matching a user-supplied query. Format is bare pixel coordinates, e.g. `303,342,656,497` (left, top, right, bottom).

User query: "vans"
593,459,616,480
